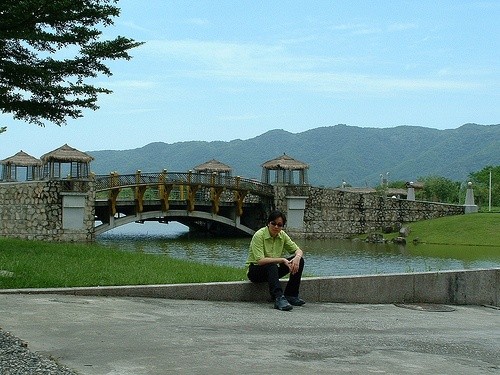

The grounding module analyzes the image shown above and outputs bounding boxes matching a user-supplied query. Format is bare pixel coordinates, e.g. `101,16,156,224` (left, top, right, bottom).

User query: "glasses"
270,221,283,227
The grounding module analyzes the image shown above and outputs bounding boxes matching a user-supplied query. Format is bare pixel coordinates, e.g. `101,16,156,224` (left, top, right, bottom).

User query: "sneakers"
286,296,305,305
274,295,293,310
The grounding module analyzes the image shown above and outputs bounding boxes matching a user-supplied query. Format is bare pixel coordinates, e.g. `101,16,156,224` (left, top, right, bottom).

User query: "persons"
246,212,306,311
